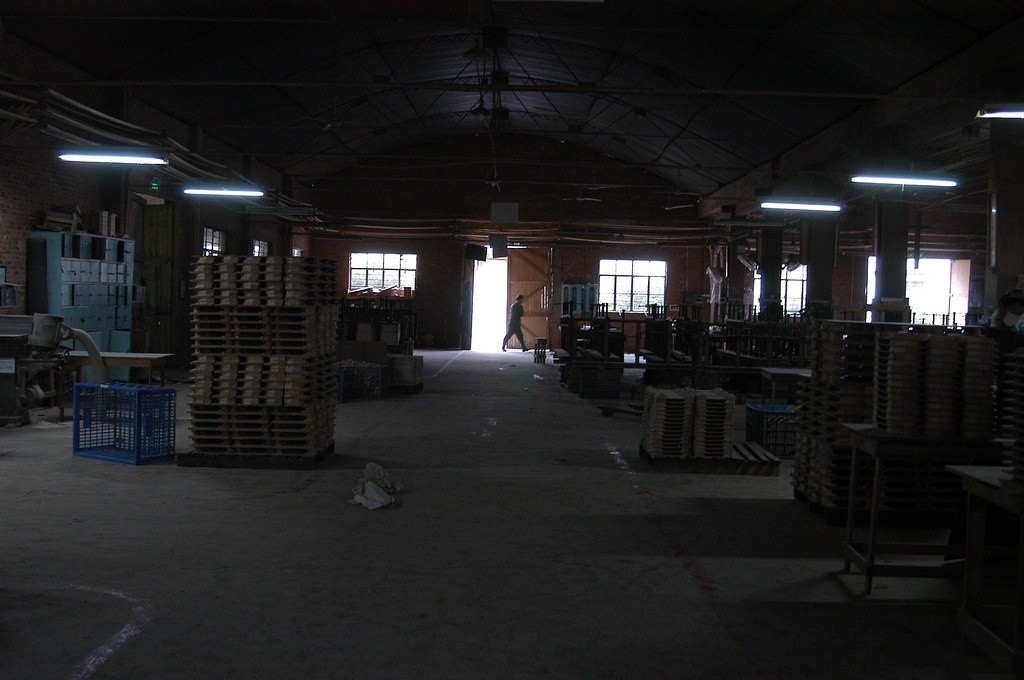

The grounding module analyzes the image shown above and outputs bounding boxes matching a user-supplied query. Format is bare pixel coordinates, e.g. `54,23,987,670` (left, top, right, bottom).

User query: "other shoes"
502,348,506,351
522,347,530,352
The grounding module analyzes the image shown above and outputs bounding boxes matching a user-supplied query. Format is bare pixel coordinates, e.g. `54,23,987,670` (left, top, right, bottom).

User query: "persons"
502,295,531,353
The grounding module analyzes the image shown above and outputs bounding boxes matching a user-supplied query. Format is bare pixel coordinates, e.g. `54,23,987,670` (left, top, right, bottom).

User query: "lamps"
57,150,169,165
485,164,502,188
463,40,492,64
471,92,490,121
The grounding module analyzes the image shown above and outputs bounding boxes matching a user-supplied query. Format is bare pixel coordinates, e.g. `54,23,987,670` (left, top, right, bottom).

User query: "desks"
57,351,174,405
838,422,1024,680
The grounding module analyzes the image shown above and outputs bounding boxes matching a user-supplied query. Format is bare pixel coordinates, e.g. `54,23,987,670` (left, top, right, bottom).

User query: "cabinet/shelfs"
27,231,145,389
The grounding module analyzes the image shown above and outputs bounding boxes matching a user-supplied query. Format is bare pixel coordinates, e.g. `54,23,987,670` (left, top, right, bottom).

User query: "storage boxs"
33,210,118,235
73,383,177,464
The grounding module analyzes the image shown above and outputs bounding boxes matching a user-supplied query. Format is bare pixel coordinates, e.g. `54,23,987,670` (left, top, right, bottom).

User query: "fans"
585,169,610,191
561,188,601,202
300,89,358,129
654,194,696,210
